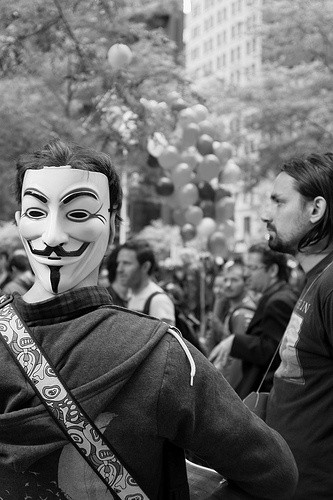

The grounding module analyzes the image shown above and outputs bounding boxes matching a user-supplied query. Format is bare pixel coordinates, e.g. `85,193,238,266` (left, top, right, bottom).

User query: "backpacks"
143,291,209,356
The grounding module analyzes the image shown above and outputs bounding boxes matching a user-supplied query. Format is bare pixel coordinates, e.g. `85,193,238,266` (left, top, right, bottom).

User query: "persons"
0,241,300,403
262,150,333,500
0,137,299,500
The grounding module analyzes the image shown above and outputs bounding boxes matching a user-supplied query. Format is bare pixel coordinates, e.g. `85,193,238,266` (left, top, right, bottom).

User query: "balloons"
108,43,132,71
157,104,245,255
